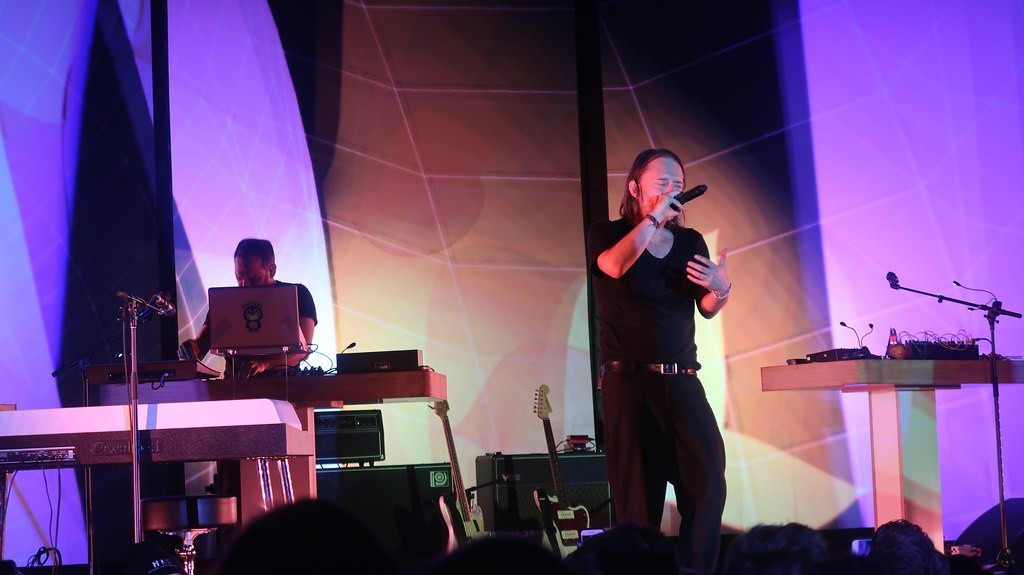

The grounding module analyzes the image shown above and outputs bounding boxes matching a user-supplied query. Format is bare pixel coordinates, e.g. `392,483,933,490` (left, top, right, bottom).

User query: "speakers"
476,454,613,538
315,461,455,552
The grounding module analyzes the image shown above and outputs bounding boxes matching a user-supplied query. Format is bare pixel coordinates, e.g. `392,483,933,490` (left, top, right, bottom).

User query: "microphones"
340,343,356,353
140,290,172,326
670,184,707,213
153,295,177,316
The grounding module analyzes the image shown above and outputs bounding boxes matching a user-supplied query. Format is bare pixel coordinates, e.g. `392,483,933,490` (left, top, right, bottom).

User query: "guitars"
433,400,485,556
532,384,591,561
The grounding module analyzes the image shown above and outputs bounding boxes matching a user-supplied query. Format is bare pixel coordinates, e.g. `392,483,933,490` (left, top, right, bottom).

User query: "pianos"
0,399,315,471
97,369,447,407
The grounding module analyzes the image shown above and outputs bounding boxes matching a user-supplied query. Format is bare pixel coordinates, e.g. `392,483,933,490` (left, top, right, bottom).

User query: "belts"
599,362,697,377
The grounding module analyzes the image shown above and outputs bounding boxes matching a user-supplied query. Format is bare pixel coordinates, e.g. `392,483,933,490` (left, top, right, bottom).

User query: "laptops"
208,285,303,346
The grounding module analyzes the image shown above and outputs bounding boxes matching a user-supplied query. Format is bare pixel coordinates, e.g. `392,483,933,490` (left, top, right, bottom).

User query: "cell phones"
580,528,606,545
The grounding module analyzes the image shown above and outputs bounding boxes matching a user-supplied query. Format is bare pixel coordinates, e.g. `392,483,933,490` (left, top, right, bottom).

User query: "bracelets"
645,214,659,229
714,285,731,299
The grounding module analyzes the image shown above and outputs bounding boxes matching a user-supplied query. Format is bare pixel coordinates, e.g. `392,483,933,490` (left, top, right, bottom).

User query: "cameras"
849,537,872,557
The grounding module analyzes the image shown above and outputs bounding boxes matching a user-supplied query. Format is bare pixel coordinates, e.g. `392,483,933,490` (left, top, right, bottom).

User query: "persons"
598,521,680,575
178,239,318,495
212,499,404,575
722,524,829,575
586,149,726,575
867,521,950,575
